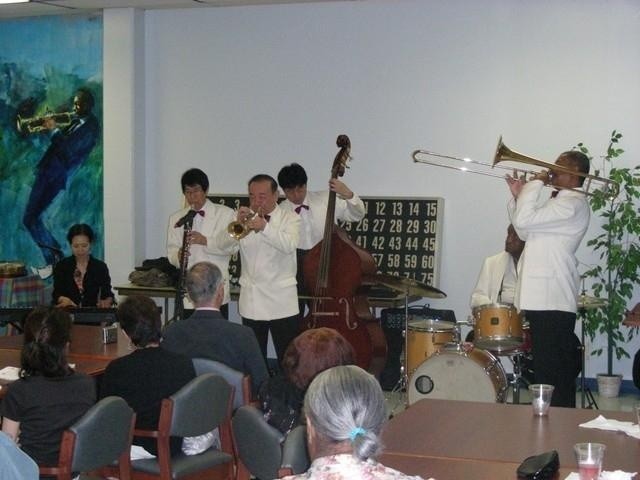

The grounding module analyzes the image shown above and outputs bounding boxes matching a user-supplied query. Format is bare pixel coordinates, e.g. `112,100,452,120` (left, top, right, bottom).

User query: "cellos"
300,134,387,382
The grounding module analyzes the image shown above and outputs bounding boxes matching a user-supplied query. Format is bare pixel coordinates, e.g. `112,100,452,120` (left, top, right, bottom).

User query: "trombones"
412,136,619,200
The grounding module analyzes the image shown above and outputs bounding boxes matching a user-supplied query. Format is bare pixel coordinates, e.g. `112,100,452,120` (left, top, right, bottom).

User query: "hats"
281,327,358,392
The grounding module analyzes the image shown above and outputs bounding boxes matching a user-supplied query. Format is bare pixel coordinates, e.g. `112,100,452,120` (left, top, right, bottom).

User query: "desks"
1,316,176,401
341,397,640,479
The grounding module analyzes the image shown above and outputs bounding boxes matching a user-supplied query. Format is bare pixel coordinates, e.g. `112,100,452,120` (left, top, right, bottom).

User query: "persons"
52,223,115,310
468,219,525,312
216,172,304,362
503,150,593,407
270,162,366,320
1,260,424,480
167,168,241,323
21,85,101,266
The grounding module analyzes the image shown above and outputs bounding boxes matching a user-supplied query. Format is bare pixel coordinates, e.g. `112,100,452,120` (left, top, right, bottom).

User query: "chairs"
39,363,308,479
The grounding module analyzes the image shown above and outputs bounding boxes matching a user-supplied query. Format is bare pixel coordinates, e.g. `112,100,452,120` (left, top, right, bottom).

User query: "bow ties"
295,205,309,213
552,191,558,197
259,214,270,222
196,210,204,217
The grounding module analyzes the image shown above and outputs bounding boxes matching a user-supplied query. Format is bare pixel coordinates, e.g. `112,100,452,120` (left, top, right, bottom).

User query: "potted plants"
570,128,640,398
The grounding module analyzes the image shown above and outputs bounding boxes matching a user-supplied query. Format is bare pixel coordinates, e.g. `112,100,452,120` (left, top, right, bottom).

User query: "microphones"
173,209,197,229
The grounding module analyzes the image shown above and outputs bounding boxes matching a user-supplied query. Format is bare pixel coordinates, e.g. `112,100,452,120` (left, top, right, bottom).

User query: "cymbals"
367,274,446,298
576,296,610,307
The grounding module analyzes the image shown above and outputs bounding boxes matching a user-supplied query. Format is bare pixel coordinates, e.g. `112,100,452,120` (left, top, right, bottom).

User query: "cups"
528,384,557,416
574,443,606,480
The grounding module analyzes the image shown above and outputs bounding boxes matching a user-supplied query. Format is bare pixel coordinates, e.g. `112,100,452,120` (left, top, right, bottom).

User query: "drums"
407,347,508,409
470,304,532,356
401,319,457,383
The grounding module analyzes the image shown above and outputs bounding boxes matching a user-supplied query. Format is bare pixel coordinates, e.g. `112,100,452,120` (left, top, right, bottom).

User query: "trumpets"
16,112,77,132
228,204,263,239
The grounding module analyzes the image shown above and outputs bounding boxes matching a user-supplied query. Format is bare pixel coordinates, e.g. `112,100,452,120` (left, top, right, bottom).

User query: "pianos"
0,305,118,323
